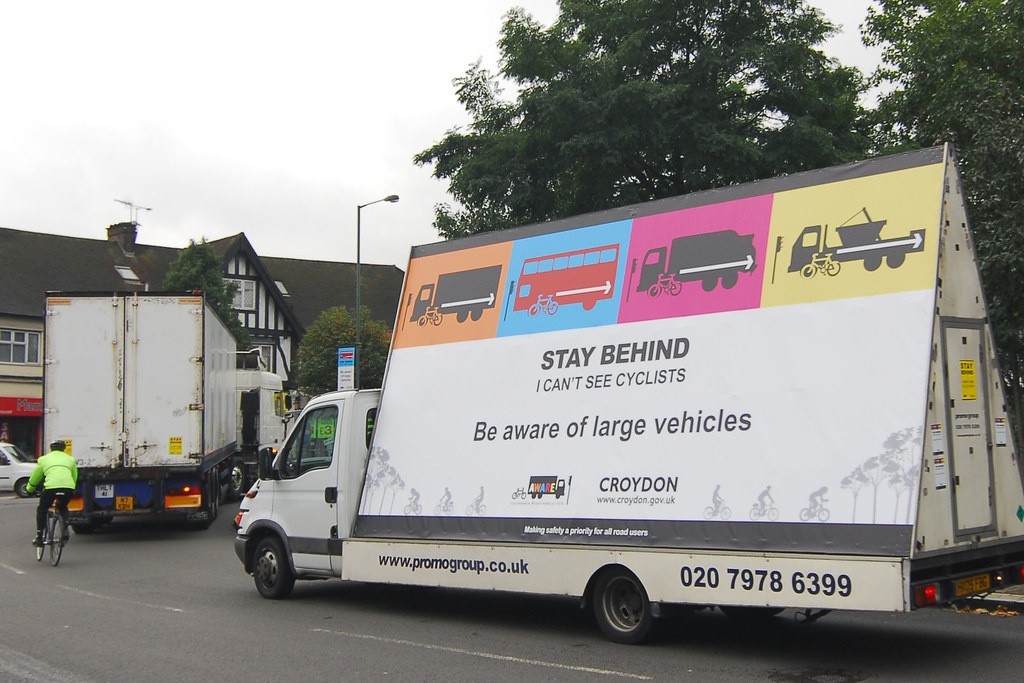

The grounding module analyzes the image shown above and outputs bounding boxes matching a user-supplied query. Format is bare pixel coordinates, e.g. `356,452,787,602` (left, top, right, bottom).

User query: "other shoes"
32,532,42,544
62,528,70,540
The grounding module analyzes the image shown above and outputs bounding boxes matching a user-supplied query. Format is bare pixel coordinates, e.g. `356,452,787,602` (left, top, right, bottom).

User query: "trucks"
234,138,1023,646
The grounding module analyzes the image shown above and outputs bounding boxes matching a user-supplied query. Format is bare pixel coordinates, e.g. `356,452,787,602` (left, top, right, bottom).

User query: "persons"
26,440,78,545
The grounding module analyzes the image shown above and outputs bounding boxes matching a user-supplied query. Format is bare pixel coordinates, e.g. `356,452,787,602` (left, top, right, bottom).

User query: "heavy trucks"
43,288,295,535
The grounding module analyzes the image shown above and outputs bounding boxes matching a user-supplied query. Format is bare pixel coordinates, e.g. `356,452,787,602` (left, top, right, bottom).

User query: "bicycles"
28,487,69,568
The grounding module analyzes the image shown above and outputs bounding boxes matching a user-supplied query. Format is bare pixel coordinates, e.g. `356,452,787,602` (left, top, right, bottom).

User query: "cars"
1,443,40,498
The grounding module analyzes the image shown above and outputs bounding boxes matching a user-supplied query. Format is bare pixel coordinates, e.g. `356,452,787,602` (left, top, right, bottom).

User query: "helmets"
49,439,66,451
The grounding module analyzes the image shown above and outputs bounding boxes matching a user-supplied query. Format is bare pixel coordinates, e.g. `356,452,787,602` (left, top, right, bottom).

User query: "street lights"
357,195,398,391
